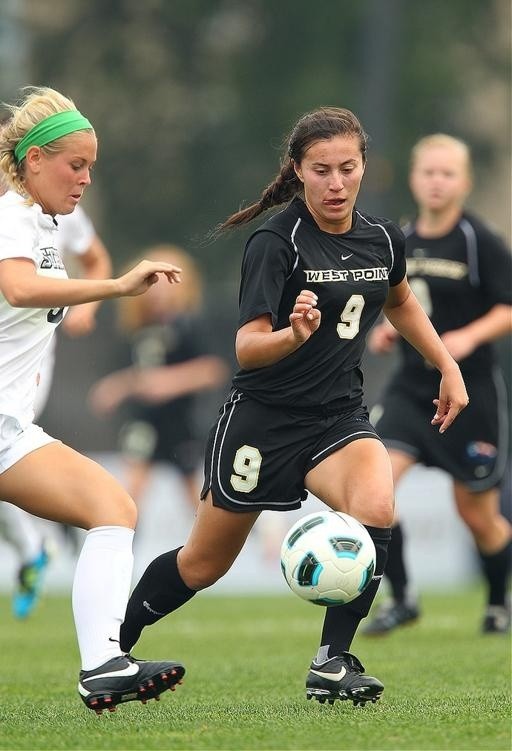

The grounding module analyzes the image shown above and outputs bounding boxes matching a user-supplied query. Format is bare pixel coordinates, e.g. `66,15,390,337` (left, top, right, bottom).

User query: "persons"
119,107,471,706
0,501,49,619
359,131,512,635
88,245,226,514
0,86,186,713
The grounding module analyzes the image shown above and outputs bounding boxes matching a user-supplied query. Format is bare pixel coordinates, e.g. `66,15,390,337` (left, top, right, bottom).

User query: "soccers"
279,513,377,606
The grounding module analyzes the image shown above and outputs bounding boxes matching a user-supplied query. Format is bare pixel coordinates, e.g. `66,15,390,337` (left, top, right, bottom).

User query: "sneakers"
75,652,186,717
303,649,385,710
480,604,510,635
359,599,420,636
